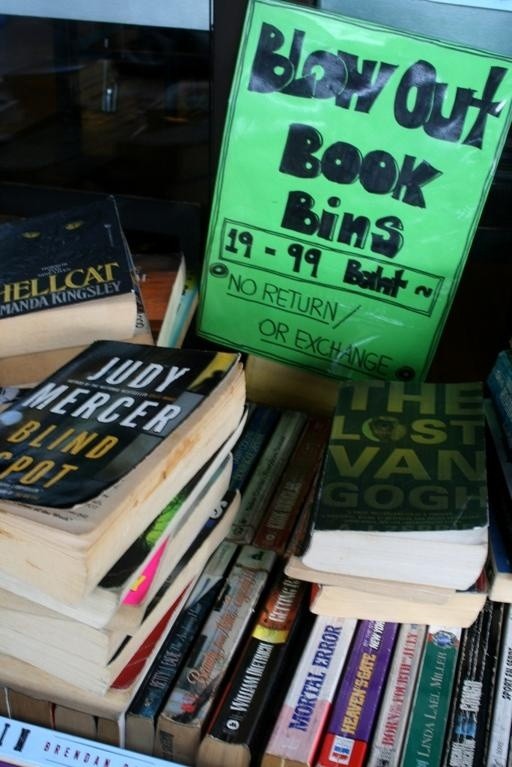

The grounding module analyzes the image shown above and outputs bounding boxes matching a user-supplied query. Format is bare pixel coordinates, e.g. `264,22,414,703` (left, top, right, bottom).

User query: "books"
0,195,145,366
309,568,488,636
1,340,310,766
301,379,489,591
310,628,512,767
135,251,199,348
284,438,492,615
473,347,512,628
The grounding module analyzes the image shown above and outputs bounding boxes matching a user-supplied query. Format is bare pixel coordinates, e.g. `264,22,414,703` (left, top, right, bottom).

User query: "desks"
1,102,212,272
